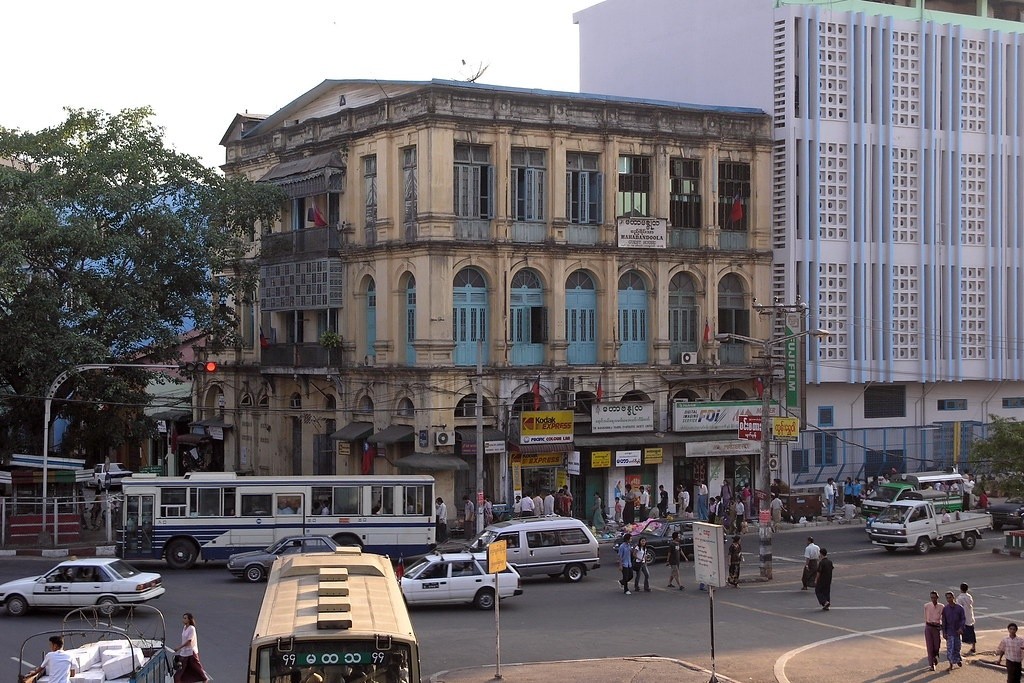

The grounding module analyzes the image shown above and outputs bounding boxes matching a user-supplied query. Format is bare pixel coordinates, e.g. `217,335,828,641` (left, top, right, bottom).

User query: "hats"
715,496,722,501
892,467,897,473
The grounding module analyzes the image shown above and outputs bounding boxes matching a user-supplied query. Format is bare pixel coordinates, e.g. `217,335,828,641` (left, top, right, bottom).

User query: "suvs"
400,550,524,610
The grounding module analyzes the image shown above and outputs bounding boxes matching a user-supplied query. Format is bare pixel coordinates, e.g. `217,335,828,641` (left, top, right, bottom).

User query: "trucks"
861,470,964,525
869,489,993,555
18,603,166,683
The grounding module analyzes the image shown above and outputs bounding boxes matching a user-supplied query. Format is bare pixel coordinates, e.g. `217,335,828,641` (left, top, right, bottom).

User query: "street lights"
714,329,830,579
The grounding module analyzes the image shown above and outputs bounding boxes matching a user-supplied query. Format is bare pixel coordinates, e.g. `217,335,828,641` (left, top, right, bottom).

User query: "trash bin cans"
779,492,822,523
493,503,509,521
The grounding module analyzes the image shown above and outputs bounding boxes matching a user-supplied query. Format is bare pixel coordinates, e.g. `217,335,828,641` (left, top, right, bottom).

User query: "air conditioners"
679,351,697,364
560,393,576,408
769,457,779,471
695,398,711,401
670,398,688,406
434,431,455,445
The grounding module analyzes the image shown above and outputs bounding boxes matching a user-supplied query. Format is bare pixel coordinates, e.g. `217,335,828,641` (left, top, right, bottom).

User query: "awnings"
395,453,469,471
660,368,770,380
367,424,415,444
456,428,505,455
151,410,233,447
330,422,373,442
508,431,775,455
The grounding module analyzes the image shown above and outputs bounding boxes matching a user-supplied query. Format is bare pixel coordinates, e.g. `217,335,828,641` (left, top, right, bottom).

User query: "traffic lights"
186,361,217,373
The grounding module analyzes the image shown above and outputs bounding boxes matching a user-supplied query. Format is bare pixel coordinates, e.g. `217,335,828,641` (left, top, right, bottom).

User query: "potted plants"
319,330,341,350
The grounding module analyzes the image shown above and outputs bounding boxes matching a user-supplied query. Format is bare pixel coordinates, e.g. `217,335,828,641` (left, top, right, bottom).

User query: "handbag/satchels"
632,559,643,571
740,555,745,561
742,522,748,533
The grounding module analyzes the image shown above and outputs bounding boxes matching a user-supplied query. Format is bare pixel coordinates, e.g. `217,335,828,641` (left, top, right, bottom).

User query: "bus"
247,546,420,683
114,471,438,570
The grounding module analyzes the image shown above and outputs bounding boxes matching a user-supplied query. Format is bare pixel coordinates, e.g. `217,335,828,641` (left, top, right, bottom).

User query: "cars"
227,534,341,583
81,463,133,491
0,556,166,618
985,491,1024,529
612,516,727,565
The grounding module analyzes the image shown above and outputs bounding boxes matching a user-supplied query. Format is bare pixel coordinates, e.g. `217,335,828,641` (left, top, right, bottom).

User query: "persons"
923,582,976,671
618,531,744,595
996,623,1024,683
270,645,409,683
29,636,79,683
48,454,788,535
174,613,208,683
825,461,1024,526
802,536,834,610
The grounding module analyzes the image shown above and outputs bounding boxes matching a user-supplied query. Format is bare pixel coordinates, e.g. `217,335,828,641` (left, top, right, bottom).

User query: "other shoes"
635,588,640,590
679,586,686,590
801,587,807,590
826,607,830,610
823,602,831,609
771,526,775,533
735,582,740,587
931,665,935,670
644,588,652,591
726,581,731,585
957,662,962,667
970,649,975,655
625,590,632,594
617,580,624,589
667,584,675,587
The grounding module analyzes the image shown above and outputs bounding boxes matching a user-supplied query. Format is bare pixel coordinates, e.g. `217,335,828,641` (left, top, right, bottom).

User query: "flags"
597,375,602,402
171,426,177,453
753,377,766,399
704,320,709,343
533,376,540,411
363,438,375,475
311,199,328,226
260,325,271,349
729,190,742,223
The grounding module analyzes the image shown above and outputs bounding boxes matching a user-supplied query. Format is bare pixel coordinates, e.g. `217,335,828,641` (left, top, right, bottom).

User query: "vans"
461,514,601,582
